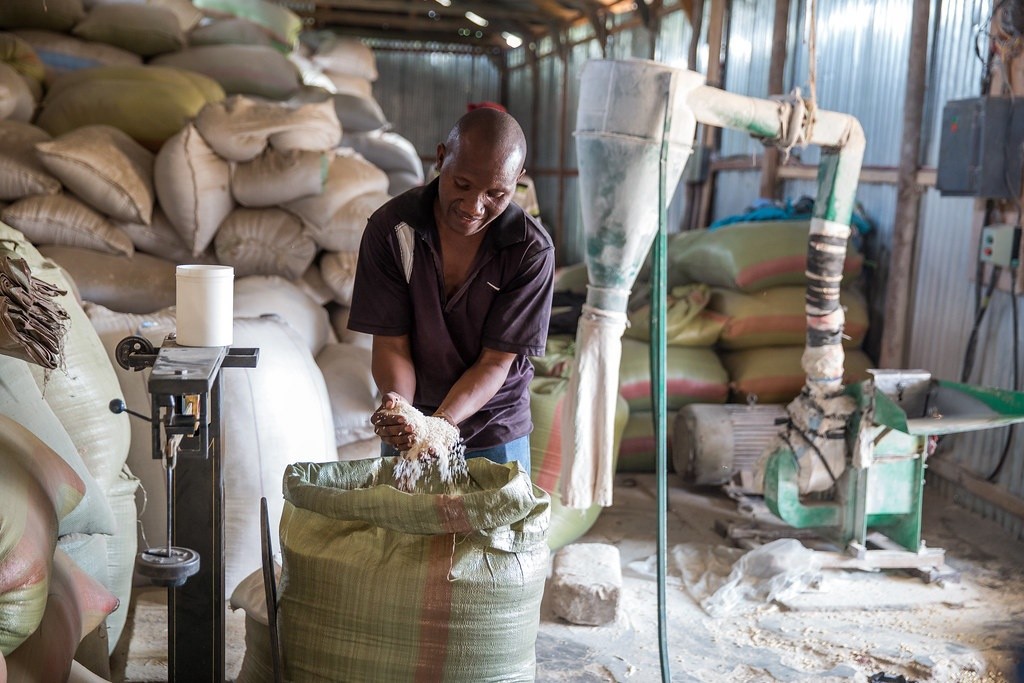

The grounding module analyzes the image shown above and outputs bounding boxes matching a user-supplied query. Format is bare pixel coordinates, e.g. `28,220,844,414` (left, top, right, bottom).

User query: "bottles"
174,264,234,348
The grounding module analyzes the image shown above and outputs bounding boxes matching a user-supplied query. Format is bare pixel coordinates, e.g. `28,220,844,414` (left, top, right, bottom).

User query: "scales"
109,330,259,683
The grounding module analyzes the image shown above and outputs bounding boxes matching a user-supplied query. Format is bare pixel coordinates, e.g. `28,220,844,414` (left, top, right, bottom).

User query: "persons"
347,109,555,479
